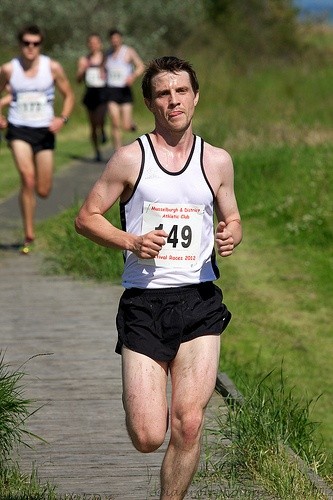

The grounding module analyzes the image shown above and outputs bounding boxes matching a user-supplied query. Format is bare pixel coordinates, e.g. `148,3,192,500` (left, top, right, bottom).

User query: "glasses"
21,40,41,47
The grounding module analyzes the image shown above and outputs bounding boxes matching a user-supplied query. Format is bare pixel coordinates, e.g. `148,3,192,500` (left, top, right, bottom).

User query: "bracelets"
59,114,69,124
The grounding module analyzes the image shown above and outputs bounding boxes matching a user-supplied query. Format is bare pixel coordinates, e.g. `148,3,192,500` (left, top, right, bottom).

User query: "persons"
74,53,244,500
103,28,145,152
75,32,108,163
0,25,75,256
